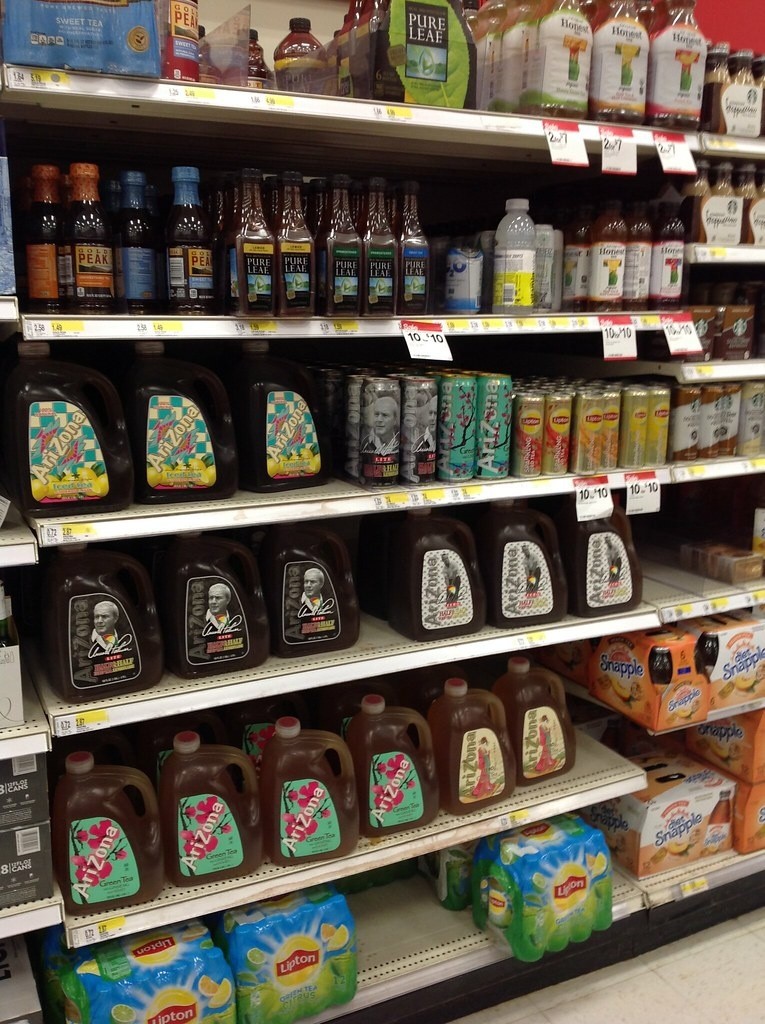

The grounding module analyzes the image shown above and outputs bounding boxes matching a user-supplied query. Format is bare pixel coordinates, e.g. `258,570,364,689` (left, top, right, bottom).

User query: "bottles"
489,199,537,316
429,678,516,816
273,17,328,95
493,657,578,787
40,494,642,706
346,692,440,837
157,731,265,888
259,716,360,868
222,28,275,90
2,341,331,520
561,160,765,312
333,0,765,139
52,749,164,917
197,25,221,85
14,162,427,316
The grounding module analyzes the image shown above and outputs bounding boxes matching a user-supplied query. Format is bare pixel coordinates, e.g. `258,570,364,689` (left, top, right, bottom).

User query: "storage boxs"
0,753,49,828
0,820,52,909
0,619,24,730
672,609,765,711
688,753,765,855
583,748,737,878
534,637,600,687
588,625,710,733
686,708,764,786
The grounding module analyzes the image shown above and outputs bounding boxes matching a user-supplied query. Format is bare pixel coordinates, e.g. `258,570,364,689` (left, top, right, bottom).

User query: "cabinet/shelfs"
0,62,700,1024
0,67,64,1024
612,134,765,949
